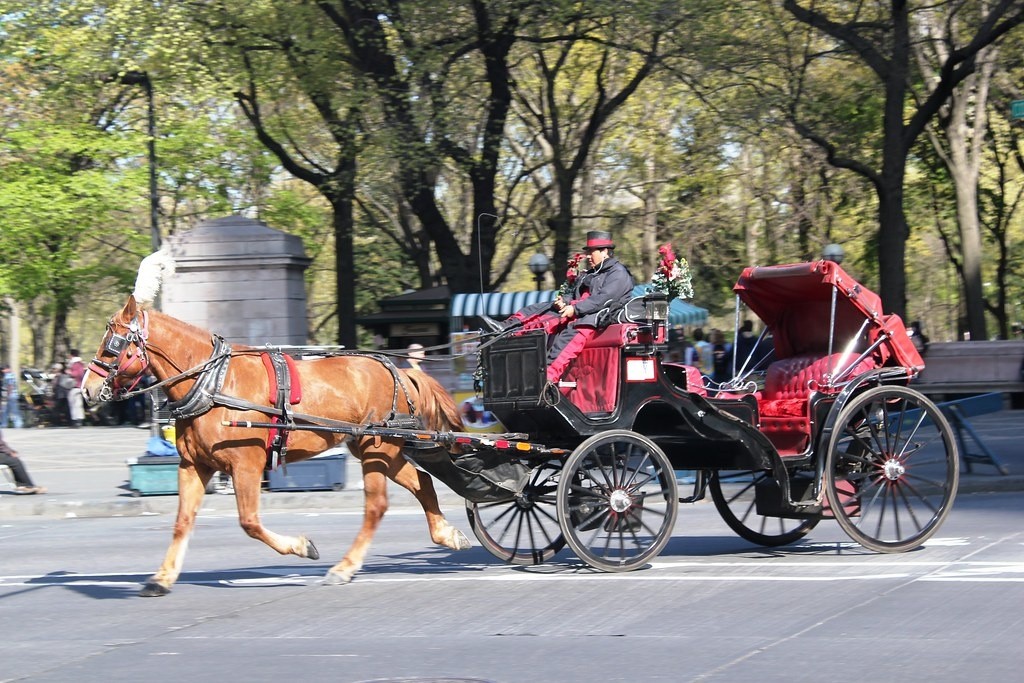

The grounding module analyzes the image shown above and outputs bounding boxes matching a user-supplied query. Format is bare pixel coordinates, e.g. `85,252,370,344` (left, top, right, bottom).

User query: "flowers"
559,253,588,296
651,244,693,303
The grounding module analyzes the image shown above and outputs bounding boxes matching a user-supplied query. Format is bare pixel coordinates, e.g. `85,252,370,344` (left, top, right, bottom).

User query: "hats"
50,363,65,371
582,231,616,250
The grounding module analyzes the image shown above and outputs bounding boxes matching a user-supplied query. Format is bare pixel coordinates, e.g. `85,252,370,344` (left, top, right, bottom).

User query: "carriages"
79,259,961,599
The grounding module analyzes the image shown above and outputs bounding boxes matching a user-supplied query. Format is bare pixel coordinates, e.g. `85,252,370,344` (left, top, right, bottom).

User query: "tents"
452,283,708,330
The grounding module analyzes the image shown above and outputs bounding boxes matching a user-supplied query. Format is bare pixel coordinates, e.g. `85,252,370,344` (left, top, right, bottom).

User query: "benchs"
541,323,664,414
660,352,876,456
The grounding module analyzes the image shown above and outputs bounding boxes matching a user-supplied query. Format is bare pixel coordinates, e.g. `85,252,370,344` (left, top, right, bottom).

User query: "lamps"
646,287,667,340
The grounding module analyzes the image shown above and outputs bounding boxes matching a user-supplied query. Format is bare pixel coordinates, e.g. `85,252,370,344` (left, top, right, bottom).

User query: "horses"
80,295,471,597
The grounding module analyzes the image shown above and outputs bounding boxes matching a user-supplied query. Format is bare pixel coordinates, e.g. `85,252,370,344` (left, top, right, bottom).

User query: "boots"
474,313,522,337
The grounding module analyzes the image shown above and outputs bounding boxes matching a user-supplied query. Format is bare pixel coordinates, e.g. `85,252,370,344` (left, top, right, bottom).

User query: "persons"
664,319,761,395
400,344,426,371
0,434,40,494
0,349,158,428
908,321,930,358
477,231,633,385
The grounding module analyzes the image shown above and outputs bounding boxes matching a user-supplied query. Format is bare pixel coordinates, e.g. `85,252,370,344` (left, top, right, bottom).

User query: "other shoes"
70,422,83,429
16,486,39,495
30,484,47,494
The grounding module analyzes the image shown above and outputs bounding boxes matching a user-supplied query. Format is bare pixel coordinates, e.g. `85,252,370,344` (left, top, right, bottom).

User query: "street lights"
107,68,161,255
